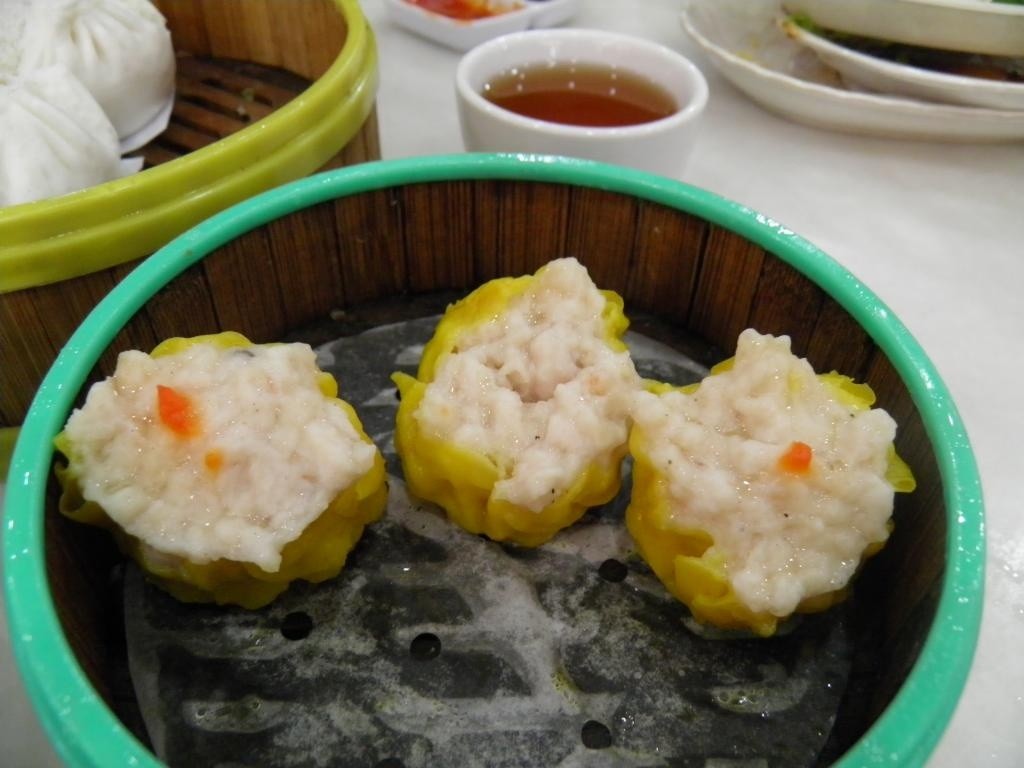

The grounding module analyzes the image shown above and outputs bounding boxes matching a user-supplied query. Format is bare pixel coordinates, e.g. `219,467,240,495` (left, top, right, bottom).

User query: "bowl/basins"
453,28,710,181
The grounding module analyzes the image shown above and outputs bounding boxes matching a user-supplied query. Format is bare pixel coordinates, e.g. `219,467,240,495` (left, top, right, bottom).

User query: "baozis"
0,0,175,206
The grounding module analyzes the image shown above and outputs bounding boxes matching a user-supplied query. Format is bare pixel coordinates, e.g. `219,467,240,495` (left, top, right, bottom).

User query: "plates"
524,0,575,29
777,14,1024,112
678,0,1024,140
384,0,538,51
780,0,1024,57
